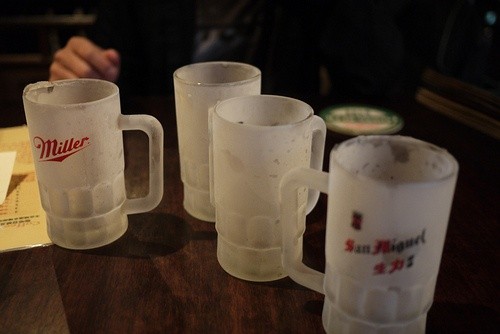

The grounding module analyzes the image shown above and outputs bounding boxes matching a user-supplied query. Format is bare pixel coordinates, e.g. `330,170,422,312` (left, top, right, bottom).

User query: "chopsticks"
416,88,500,138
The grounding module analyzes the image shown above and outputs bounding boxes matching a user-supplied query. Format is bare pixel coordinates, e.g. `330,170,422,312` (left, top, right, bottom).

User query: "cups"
212,94,327,281
280,135,460,333
173,61,262,222
22,78,164,251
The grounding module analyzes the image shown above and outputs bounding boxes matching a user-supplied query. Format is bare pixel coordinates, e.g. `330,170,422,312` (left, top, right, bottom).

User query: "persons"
47,0,320,100
318,0,479,96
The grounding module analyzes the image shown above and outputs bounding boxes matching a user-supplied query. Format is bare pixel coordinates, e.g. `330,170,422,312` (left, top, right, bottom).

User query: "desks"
0,91,500,334
